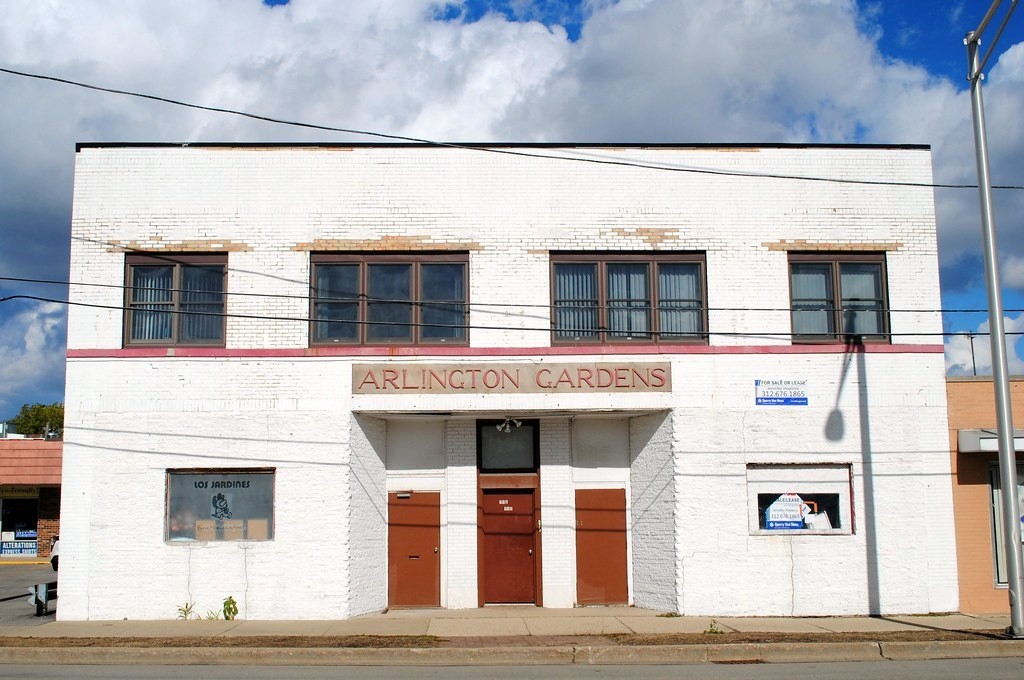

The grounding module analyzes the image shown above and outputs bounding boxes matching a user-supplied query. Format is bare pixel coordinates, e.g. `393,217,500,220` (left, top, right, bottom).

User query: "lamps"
496,416,522,433
